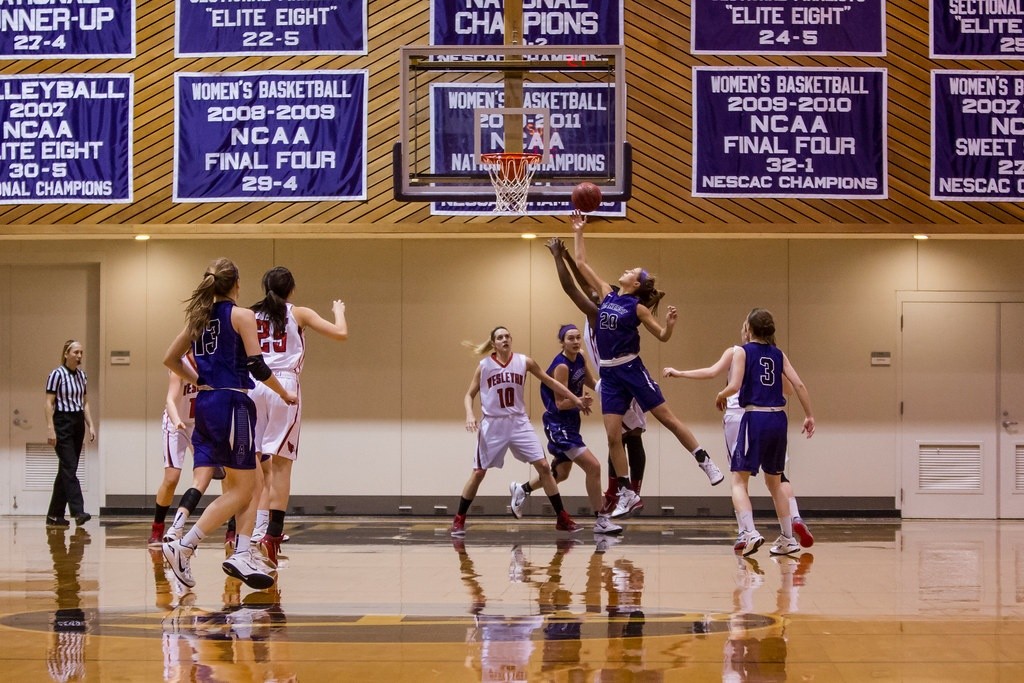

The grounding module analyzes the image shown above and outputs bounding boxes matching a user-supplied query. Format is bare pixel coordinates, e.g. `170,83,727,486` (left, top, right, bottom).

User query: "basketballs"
572,182,602,213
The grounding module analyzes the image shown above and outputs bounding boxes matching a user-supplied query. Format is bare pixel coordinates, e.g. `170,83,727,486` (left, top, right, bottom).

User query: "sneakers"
148,522,166,547
225,530,236,557
46,515,71,525
611,486,644,518
162,539,196,588
256,535,284,569
597,476,619,516
452,513,465,536
792,517,814,547
742,529,766,556
733,530,750,550
163,525,184,542
250,528,289,544
593,534,622,546
557,539,585,550
737,557,766,589
768,554,801,574
593,517,623,534
770,532,801,554
76,513,92,525
630,499,648,516
451,534,466,551
555,512,585,532
510,482,529,519
148,547,165,563
222,554,274,589
695,448,725,486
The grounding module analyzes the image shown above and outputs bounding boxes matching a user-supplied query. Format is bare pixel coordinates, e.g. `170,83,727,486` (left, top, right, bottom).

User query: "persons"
43,339,96,527
451,326,592,534
509,324,623,532
567,209,724,517
543,237,646,515
148,257,347,589
715,308,815,556
662,320,814,550
